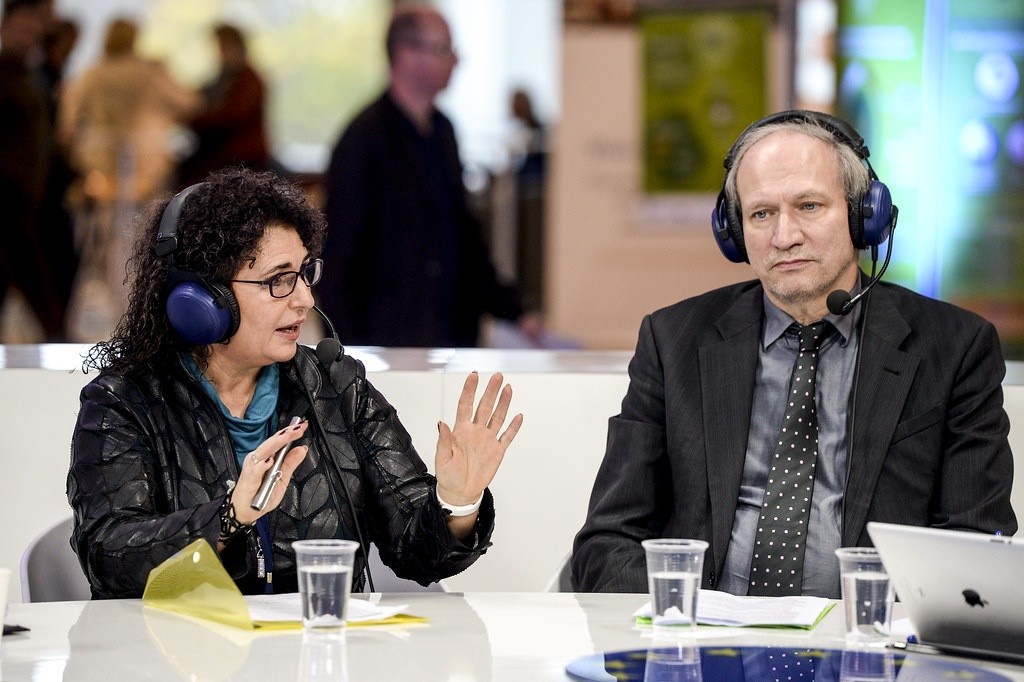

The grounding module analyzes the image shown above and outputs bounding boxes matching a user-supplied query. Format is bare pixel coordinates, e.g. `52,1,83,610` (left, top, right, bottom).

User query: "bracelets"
217,489,250,543
436,482,484,516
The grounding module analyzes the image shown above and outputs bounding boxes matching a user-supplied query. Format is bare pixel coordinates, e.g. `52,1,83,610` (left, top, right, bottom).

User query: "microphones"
826,218,897,315
311,305,344,364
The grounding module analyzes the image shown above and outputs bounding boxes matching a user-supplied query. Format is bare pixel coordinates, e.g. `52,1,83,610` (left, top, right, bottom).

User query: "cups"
641,538,709,636
643,645,702,682
834,546,895,647
840,640,897,682
0,567,13,643
291,538,361,634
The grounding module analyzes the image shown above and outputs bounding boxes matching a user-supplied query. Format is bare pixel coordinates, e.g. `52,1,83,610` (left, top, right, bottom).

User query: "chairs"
27,515,445,604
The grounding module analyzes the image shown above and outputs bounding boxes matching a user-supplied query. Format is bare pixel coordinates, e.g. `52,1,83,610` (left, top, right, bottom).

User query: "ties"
766,647,815,682
745,319,837,596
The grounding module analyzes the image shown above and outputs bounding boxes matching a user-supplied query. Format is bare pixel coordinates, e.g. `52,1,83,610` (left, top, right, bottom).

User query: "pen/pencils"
251,416,301,511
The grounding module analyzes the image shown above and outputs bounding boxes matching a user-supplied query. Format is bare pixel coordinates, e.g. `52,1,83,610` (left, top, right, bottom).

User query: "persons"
64,165,524,601
311,5,547,351
0,1,275,343
500,87,544,305
569,106,1020,600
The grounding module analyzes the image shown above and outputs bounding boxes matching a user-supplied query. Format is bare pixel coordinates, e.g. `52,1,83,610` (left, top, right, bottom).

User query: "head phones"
711,110,893,264
155,182,241,344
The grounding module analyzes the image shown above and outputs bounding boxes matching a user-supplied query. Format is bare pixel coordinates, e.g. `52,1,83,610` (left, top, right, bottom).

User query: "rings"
252,452,260,463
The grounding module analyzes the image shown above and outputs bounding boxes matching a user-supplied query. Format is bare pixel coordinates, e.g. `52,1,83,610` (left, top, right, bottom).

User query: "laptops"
866,522,1023,662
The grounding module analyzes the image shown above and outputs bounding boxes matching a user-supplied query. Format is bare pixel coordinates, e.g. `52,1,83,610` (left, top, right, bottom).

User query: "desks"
0,593,909,682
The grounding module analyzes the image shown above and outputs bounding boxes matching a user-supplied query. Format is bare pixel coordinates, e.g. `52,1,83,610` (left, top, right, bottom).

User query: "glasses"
230,259,323,299
